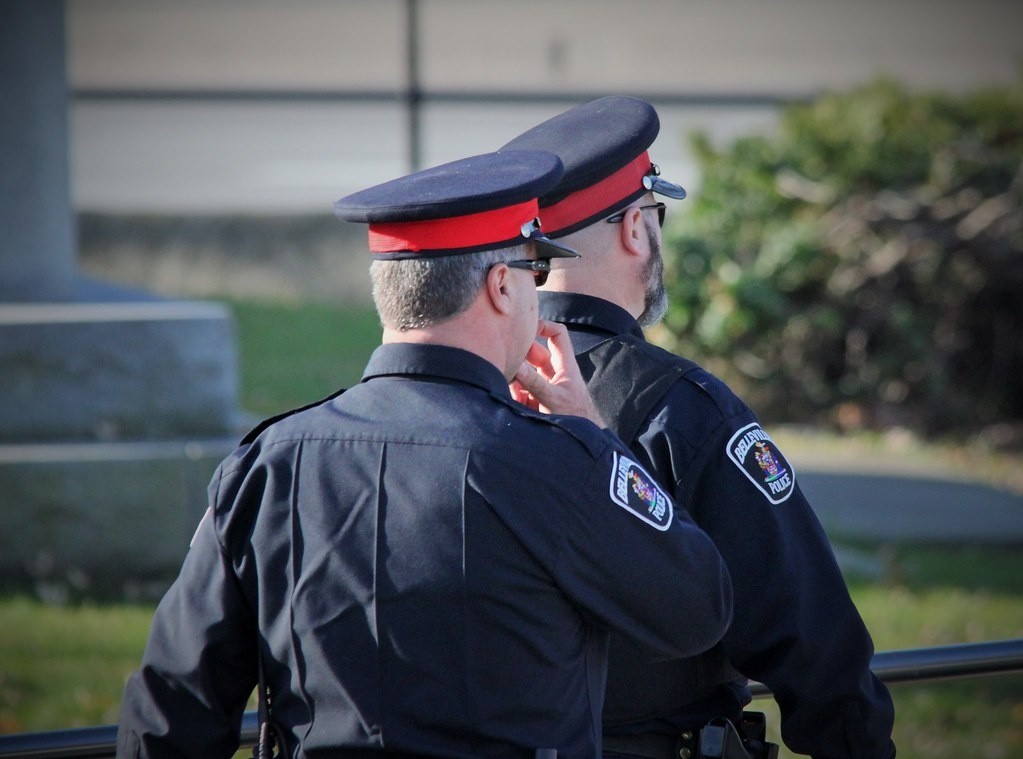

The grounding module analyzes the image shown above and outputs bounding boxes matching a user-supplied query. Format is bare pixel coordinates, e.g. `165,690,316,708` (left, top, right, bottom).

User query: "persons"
115,151,735,759
498,97,896,759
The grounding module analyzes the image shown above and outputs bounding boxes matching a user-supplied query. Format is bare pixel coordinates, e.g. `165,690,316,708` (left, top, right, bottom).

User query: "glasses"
487,258,551,287
607,202,667,228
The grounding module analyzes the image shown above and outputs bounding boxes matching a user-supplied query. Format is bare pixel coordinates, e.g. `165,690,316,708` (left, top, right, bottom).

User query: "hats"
334,152,582,262
497,96,686,239
